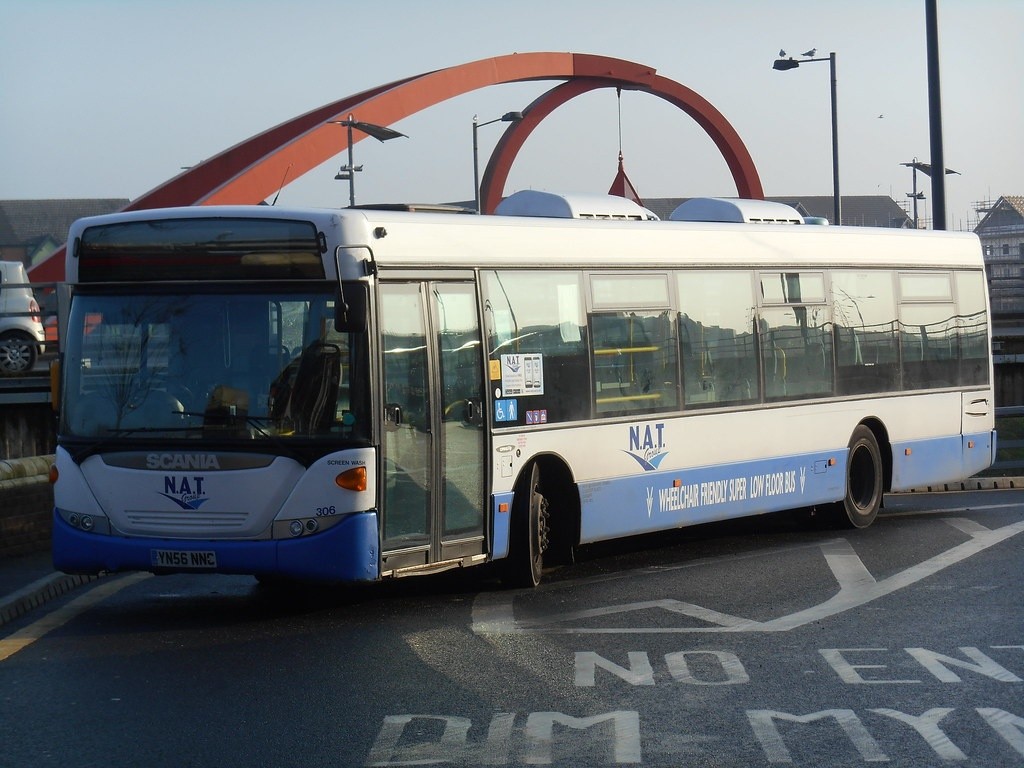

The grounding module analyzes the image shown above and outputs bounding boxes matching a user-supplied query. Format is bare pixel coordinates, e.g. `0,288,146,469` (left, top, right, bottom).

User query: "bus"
48,206,997,589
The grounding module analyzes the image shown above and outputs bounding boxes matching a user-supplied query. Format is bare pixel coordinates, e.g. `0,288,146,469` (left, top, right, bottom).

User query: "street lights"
471,111,524,214
900,158,962,229
326,109,409,205
773,50,841,226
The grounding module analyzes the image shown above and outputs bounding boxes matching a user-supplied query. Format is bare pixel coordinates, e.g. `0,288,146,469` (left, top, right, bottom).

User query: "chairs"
289,341,340,434
601,336,835,405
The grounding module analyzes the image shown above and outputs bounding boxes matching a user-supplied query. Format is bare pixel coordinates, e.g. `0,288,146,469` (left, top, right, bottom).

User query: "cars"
0,260,45,375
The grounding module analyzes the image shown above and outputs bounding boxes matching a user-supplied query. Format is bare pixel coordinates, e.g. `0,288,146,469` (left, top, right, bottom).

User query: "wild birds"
801,48,817,60
779,48,787,60
